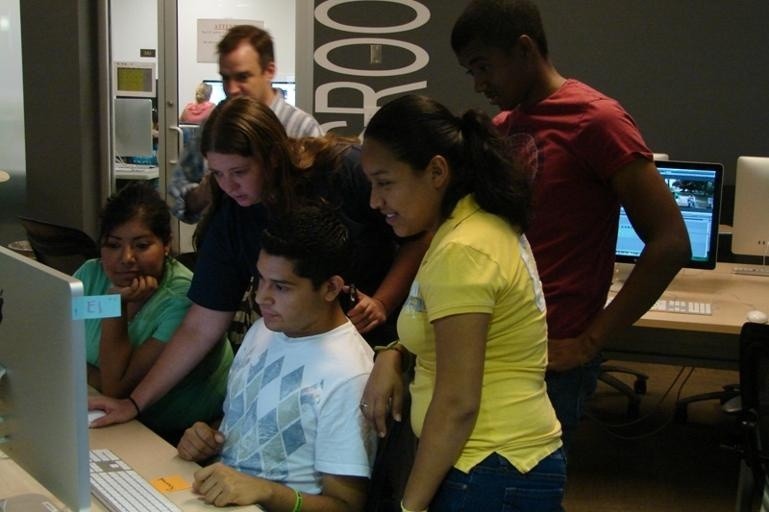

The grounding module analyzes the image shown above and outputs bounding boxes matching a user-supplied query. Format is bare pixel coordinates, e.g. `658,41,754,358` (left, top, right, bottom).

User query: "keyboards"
732,267,769,277
89,448,183,512
603,296,711,316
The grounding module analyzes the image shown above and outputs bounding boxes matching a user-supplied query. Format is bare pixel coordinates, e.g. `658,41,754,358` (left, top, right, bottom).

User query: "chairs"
738,322,768,512
674,383,740,419
596,363,649,418
13,216,102,279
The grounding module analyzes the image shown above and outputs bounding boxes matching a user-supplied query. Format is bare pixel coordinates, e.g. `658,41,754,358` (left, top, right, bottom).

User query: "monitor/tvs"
113,61,156,98
0,246,90,512
114,98,153,163
203,80,227,106
271,82,296,108
614,160,724,270
731,156,769,264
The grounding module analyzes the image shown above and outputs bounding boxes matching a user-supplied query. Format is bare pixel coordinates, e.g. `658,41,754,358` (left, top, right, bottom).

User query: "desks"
598,257,768,430
1,381,265,511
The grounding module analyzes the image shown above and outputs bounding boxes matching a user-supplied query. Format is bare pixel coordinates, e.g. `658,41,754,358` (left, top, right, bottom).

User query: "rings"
361,400,368,407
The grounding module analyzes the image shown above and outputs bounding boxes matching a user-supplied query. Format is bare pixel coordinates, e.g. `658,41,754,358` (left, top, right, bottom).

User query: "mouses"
88,410,106,428
746,309,767,324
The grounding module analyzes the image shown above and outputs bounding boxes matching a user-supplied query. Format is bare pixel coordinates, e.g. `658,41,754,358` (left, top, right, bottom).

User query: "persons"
361,95,566,511
453,2,691,452
177,217,378,511
73,186,234,442
169,24,323,224
87,96,417,511
180,88,215,124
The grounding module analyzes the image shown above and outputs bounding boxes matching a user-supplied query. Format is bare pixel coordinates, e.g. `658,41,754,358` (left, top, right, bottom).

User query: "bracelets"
400,499,428,511
294,489,303,511
127,396,141,415
